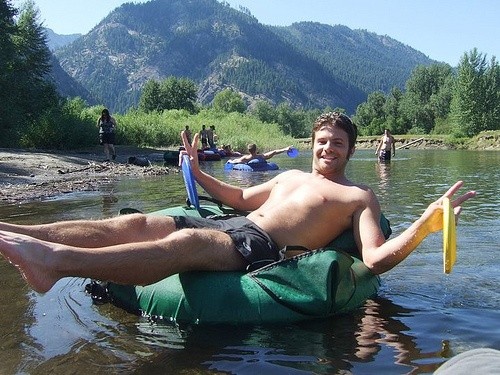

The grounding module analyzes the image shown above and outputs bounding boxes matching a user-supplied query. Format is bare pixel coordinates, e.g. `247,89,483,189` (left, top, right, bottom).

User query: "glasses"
102,114,108,116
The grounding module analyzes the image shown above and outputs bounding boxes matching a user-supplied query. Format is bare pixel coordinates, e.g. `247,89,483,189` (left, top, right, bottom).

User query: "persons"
97,109,117,162
374,164,393,197
182,124,237,156
125,295,492,375
226,143,294,164
374,128,395,164
0,110,474,295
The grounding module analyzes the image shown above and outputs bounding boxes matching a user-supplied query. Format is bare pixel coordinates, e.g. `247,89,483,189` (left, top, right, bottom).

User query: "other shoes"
112,153,117,160
103,159,110,162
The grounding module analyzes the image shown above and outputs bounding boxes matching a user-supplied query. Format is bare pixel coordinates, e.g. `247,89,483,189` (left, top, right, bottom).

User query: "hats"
210,124,216,130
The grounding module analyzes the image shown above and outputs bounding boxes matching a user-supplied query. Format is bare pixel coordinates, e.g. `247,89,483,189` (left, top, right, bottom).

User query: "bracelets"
392,153,394,154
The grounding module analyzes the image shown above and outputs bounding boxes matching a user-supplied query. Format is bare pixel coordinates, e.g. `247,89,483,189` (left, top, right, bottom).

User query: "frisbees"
442,193,458,275
287,147,298,158
179,154,200,207
224,160,233,171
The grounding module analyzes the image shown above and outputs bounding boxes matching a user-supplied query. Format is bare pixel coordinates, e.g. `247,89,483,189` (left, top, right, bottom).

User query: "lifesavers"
224,158,279,171
89,199,393,331
